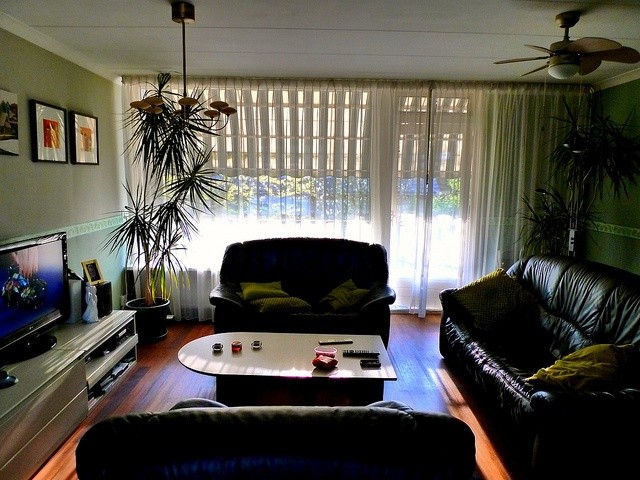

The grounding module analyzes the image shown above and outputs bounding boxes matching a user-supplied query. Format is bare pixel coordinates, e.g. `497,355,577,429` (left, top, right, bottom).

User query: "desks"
177,331,398,405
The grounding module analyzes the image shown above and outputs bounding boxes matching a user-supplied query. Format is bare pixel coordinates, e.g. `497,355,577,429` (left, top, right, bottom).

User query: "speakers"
81,278,112,318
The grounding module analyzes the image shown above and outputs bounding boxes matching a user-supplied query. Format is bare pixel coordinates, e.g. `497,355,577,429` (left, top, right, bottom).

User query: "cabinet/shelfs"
1,308,137,478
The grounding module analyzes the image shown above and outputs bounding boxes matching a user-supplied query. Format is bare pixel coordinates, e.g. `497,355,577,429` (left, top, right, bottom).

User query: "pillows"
251,297,310,311
321,278,371,311
239,280,289,301
523,342,635,391
452,267,535,328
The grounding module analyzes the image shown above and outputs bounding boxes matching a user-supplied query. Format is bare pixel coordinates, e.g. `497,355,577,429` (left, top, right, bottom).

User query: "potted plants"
106,72,228,344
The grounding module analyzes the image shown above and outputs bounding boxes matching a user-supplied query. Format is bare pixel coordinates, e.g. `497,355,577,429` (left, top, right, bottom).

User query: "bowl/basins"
314,346,338,358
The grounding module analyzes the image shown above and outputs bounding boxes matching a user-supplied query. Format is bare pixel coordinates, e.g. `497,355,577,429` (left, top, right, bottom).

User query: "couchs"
208,237,395,351
75,399,476,479
439,252,640,475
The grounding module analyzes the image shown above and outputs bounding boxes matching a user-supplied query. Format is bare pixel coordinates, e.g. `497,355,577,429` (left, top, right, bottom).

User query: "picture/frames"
82,259,104,286
0,88,19,156
28,97,69,164
69,110,100,164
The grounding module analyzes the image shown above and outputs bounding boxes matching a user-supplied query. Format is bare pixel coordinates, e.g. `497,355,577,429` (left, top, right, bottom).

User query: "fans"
493,11,639,80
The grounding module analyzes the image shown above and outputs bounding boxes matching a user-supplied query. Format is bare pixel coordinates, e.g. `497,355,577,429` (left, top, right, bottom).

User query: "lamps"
129,1,237,132
548,56,580,79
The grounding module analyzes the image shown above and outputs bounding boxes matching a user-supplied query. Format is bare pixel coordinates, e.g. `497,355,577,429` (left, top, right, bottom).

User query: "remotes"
318,339,353,345
343,350,380,357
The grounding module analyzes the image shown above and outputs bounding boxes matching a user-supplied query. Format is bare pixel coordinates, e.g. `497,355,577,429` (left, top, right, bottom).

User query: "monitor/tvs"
0,231,71,365
